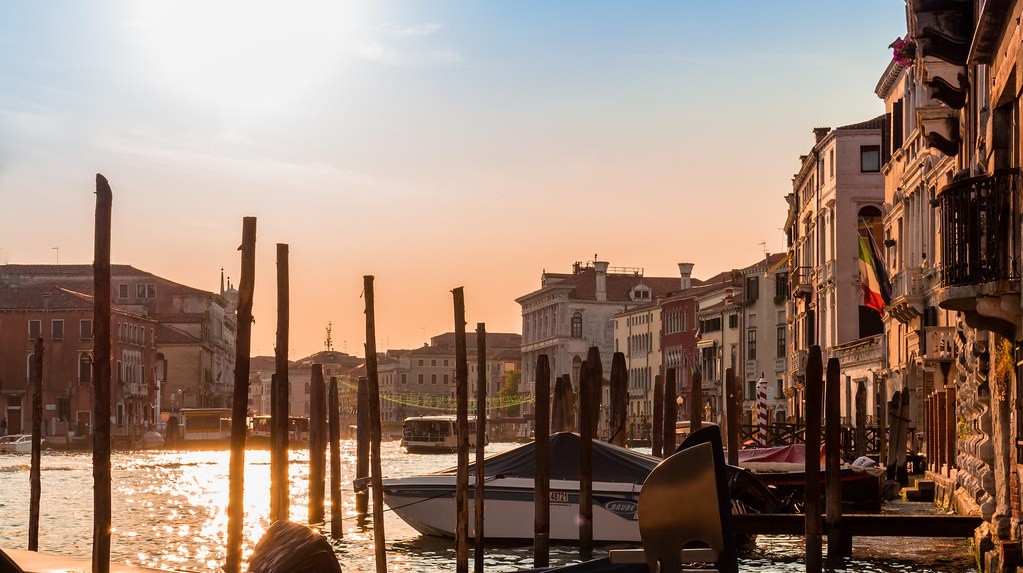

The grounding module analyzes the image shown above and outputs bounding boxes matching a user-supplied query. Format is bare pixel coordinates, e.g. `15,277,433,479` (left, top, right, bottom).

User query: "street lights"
677,396,683,422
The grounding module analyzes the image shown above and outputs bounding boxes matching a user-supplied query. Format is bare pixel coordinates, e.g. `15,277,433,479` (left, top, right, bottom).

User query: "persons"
144,419,148,431
0,417,6,437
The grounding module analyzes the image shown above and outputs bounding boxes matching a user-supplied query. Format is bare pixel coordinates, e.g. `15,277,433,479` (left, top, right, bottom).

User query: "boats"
131,430,165,450
0,434,46,454
754,469,887,514
248,415,329,450
401,415,489,454
354,429,804,545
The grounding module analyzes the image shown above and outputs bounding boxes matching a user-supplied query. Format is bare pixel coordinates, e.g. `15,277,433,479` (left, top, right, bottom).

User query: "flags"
691,366,699,376
857,229,892,317
